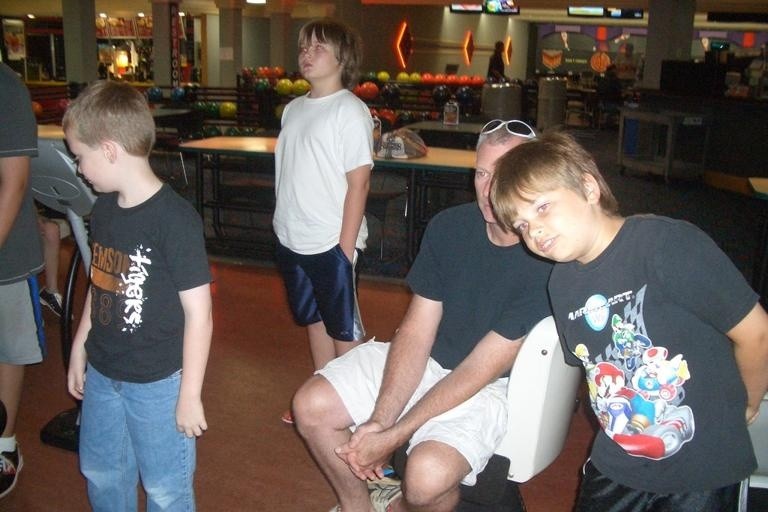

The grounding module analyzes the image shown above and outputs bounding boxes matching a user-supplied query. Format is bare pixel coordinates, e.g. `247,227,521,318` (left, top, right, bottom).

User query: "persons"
291,119,560,512
486,41,509,81
273,16,377,427
61,77,214,512
0,60,48,499
482,128,768,512
38,218,74,322
616,42,643,81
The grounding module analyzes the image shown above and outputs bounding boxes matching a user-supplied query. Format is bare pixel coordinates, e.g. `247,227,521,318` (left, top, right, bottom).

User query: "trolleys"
618,104,713,193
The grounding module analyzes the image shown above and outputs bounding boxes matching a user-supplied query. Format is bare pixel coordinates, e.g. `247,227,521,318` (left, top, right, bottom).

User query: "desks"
149,106,497,289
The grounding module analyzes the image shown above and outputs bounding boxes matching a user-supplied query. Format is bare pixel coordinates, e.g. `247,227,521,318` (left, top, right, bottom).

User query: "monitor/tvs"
708,11,768,22
485,0,520,15
567,6,605,17
450,0,484,13
606,6,643,19
660,59,703,96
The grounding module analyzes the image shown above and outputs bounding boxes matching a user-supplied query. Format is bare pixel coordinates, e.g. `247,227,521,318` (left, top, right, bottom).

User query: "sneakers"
0,441,23,500
330,477,403,512
39,288,74,321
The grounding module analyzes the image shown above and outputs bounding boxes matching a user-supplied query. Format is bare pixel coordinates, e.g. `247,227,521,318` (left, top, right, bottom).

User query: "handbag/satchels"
376,129,428,160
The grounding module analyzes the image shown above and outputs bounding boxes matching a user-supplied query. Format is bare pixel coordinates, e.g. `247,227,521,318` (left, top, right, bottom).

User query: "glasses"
475,118,536,149
